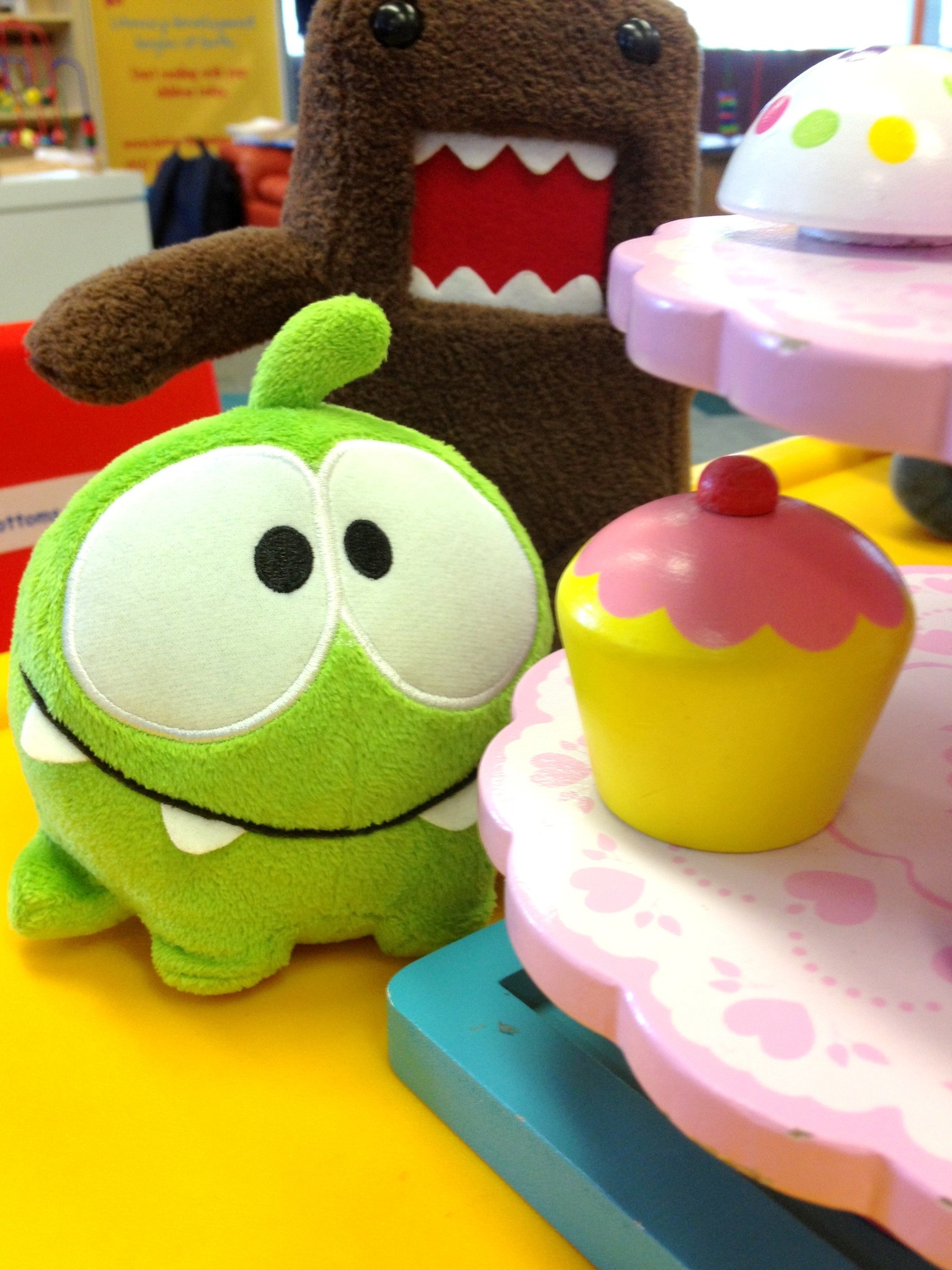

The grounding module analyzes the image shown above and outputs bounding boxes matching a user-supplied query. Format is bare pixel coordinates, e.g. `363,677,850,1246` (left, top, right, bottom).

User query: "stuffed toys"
8,293,553,995
22,0,704,651
